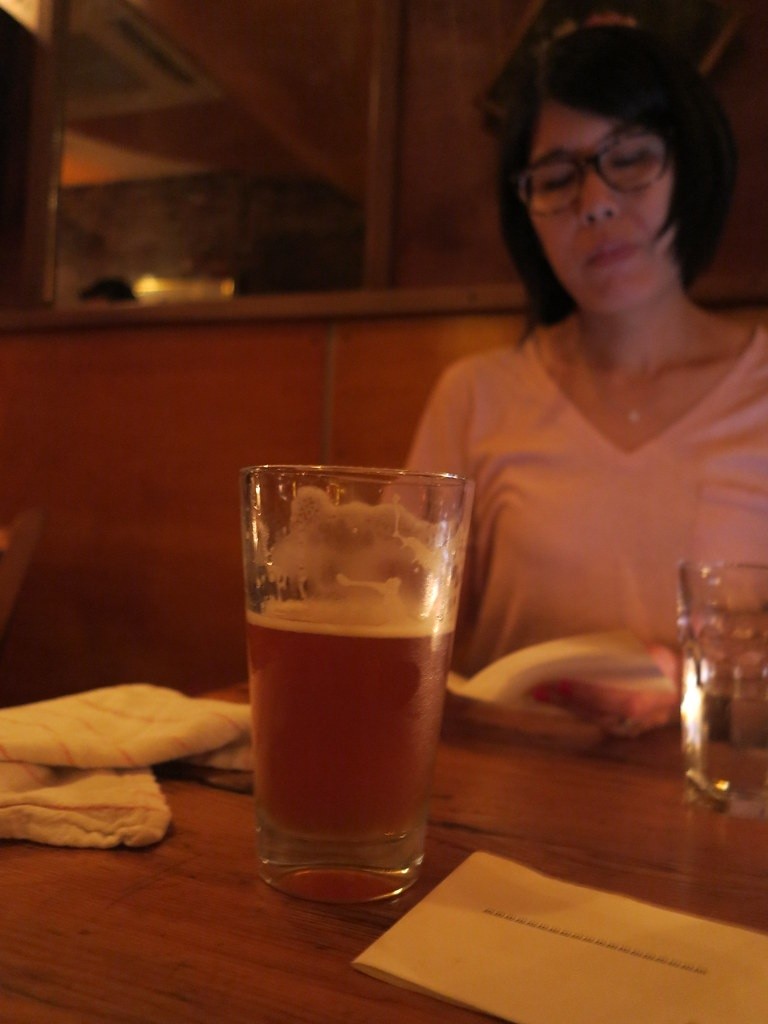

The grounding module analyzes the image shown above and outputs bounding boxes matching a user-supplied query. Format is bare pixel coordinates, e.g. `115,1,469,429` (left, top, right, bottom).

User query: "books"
443,633,665,750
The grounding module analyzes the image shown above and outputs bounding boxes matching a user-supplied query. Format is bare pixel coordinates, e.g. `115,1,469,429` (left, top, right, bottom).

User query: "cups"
672,555,768,820
236,463,477,904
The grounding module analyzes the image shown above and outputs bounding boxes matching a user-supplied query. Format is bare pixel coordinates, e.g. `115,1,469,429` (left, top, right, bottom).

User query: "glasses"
513,117,679,214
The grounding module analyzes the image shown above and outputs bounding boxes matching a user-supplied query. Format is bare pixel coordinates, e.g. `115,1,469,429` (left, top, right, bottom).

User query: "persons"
365,26,768,740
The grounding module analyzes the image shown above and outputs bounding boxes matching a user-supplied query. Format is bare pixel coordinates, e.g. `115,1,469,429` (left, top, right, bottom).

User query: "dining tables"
0,677,768,1024
